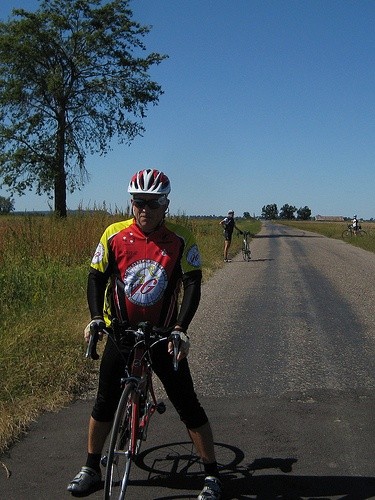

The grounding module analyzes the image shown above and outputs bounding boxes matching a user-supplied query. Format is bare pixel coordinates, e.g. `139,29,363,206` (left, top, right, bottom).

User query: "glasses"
133,198,166,210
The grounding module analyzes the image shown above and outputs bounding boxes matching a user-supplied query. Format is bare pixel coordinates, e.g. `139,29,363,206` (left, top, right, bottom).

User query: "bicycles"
84,321,186,500
342,223,367,239
236,230,252,262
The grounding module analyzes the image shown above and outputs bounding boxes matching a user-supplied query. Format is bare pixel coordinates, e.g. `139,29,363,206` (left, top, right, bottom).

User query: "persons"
66,169,222,500
352,215,358,236
218,210,243,263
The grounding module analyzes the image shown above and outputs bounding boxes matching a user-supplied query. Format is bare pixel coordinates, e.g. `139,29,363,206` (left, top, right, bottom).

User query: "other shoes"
224,259,233,262
67,466,102,492
198,472,224,500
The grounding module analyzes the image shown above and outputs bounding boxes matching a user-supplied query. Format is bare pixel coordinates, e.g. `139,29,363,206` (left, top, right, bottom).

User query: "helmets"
353,215,357,219
128,168,171,194
227,209,234,216
